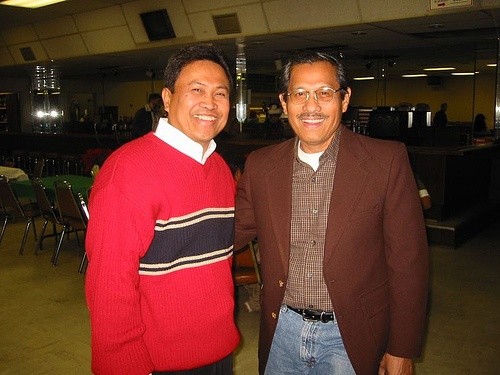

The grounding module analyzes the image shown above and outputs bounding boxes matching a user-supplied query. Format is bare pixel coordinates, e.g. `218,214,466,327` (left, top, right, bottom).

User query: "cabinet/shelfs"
0,92,17,134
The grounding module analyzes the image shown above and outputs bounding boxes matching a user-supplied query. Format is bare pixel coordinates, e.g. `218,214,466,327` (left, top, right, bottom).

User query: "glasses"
287,86,343,104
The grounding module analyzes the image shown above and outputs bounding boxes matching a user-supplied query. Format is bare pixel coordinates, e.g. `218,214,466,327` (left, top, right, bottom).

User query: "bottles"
107,113,132,136
38,118,59,135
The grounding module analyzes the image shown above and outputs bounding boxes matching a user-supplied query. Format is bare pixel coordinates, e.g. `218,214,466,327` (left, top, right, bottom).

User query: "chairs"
0,177,88,274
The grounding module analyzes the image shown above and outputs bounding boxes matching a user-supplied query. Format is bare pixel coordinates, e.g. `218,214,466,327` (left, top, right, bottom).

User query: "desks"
10,174,95,241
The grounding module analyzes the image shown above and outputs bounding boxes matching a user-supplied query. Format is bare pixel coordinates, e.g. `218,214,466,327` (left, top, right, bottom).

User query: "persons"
430,102,448,129
84,45,243,375
232,50,433,375
129,91,162,142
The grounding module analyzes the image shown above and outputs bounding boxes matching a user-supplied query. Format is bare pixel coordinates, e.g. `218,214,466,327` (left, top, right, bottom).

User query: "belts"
284,303,335,323
